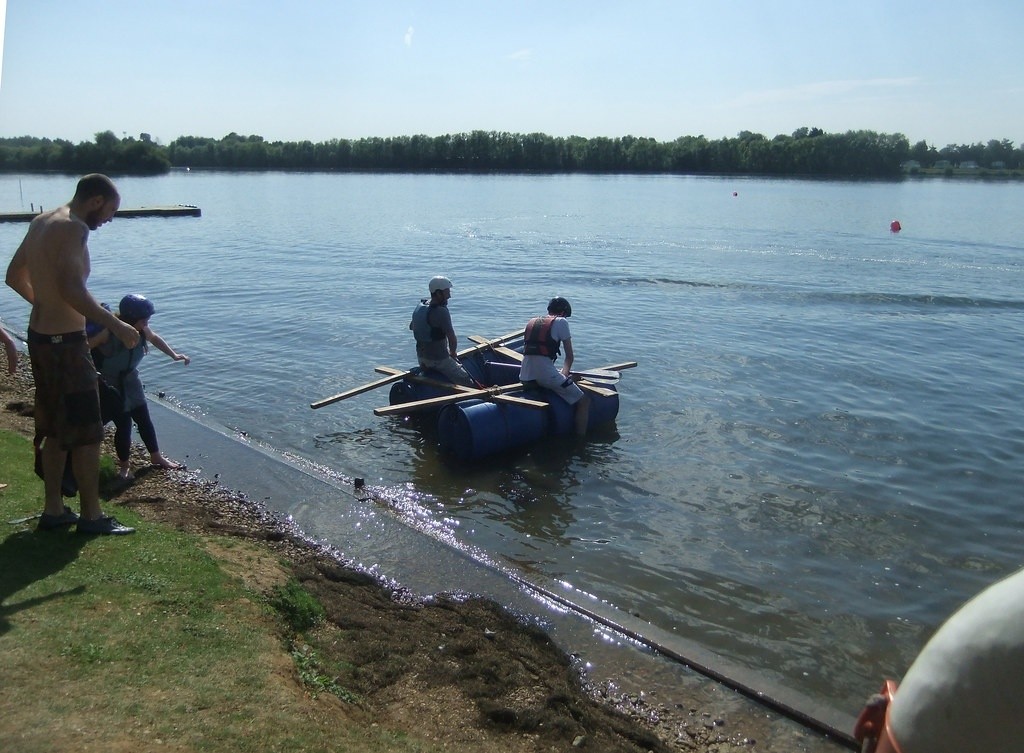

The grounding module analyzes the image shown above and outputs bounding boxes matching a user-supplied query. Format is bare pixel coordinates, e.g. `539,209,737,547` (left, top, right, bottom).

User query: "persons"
408,276,474,387
89,294,191,477
4,172,139,536
519,297,590,439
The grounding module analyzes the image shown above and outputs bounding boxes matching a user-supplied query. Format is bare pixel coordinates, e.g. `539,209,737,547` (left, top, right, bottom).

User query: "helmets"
119,294,155,324
428,277,452,294
548,296,572,318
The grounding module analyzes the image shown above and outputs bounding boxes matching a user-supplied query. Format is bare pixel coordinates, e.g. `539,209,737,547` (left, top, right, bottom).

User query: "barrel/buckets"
436,379,619,461
389,340,528,419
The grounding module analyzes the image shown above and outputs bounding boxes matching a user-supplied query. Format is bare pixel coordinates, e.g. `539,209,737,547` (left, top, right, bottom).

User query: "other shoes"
77,513,135,535
40,507,80,526
